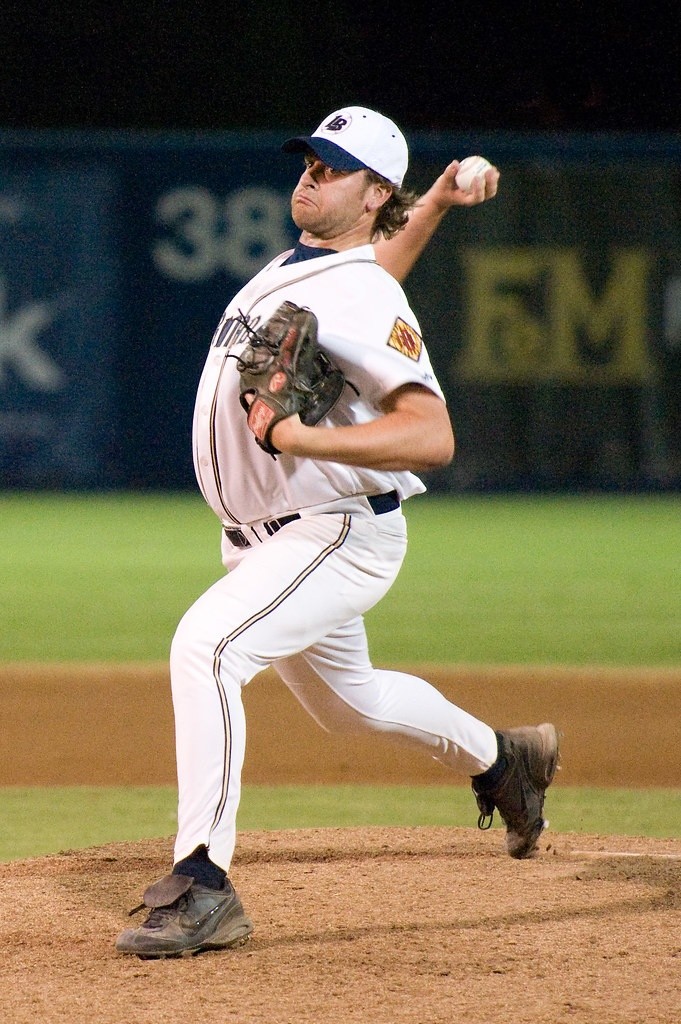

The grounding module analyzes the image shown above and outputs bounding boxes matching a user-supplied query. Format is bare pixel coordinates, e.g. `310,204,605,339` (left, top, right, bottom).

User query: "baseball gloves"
234,297,350,458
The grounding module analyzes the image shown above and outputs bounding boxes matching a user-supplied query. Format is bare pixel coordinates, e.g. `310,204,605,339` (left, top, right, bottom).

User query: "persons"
117,106,559,961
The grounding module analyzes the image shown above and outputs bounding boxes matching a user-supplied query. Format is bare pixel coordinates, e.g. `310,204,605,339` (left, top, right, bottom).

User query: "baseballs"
452,152,498,196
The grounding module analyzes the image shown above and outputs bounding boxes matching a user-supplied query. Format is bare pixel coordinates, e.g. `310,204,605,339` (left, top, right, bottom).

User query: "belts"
224,489,400,547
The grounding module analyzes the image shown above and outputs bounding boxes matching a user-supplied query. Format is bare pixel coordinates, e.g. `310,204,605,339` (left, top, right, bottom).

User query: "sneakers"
116,874,253,955
471,723,558,860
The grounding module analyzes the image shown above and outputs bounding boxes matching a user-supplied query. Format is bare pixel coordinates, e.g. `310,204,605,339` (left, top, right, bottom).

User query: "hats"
281,106,408,191
